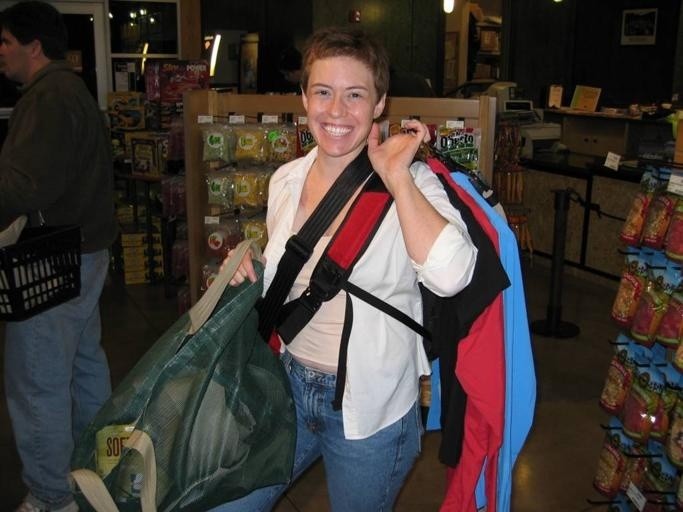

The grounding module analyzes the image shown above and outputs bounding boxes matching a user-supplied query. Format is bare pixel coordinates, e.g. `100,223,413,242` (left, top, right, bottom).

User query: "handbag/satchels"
67,237,297,512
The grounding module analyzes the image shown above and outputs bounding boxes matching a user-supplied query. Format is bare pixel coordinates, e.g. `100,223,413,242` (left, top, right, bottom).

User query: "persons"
208,24,479,512
0,1,119,512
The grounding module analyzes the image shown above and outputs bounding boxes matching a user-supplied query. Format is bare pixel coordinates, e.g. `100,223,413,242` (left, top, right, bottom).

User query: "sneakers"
14,491,80,512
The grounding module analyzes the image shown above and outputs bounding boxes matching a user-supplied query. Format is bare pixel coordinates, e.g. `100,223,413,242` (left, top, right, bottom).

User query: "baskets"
0,228,81,323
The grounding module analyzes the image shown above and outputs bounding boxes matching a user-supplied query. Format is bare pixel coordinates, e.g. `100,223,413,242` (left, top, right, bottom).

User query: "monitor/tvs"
504,100,533,111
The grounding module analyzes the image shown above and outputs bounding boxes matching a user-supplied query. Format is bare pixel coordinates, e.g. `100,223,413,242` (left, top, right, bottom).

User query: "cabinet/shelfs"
103,2,497,312
493,98,672,338
